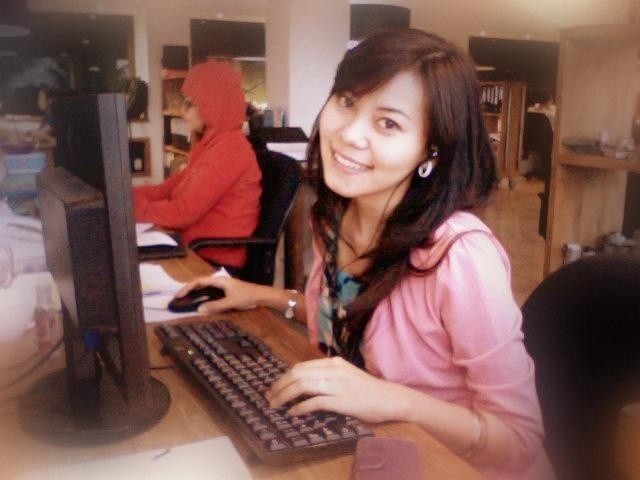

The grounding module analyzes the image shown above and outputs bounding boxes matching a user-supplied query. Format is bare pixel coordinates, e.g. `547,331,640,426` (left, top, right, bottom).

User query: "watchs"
284,288,298,321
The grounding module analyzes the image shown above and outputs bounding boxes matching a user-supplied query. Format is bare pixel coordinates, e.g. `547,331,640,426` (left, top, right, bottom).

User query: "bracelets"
459,411,484,459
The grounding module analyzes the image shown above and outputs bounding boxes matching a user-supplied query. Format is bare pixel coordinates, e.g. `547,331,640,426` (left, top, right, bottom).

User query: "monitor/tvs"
19,92,170,447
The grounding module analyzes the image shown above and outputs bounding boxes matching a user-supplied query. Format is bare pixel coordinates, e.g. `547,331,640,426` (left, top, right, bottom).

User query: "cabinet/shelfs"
542,27,640,280
480,80,527,173
158,69,194,183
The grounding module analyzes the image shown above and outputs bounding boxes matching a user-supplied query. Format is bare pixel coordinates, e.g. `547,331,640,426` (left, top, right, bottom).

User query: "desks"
0,178,482,479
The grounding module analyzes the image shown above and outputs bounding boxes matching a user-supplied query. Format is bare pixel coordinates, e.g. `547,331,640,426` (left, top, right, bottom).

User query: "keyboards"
152,317,375,466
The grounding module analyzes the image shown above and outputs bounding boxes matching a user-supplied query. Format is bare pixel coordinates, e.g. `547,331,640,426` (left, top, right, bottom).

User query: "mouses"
168,286,225,312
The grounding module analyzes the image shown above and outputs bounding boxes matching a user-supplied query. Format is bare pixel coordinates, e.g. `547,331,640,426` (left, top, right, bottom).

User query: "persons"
177,28,558,479
132,54,262,281
5,82,61,150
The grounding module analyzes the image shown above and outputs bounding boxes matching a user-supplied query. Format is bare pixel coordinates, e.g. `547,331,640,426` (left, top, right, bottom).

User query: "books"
479,83,504,113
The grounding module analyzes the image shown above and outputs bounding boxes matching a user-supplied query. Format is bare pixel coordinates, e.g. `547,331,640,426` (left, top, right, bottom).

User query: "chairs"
518,250,639,480
188,148,303,285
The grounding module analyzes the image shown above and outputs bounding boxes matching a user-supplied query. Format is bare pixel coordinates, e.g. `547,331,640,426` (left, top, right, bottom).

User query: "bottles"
29,275,62,358
